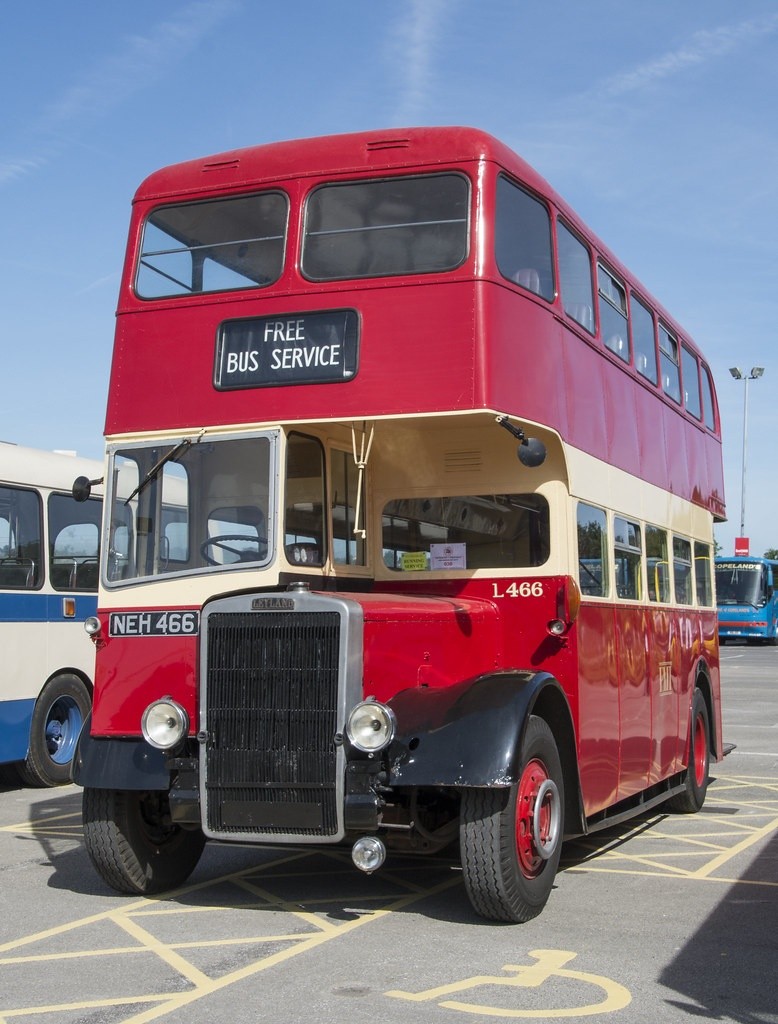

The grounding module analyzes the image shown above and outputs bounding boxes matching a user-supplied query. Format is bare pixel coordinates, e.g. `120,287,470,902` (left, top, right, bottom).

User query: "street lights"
728,365,765,556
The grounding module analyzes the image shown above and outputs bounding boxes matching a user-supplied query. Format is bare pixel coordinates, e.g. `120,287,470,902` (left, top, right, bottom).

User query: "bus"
0,440,223,789
67,125,736,925
711,555,778,645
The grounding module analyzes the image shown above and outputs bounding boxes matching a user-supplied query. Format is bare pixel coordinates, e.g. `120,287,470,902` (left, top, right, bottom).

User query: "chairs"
511,268,689,409
0,556,99,589
